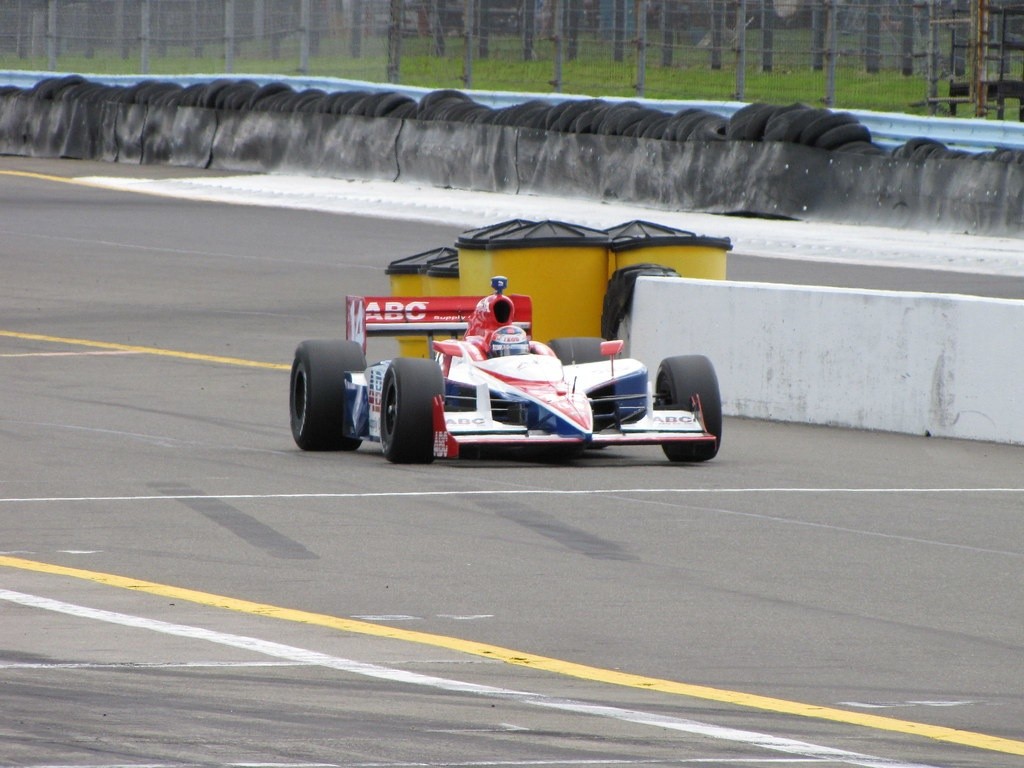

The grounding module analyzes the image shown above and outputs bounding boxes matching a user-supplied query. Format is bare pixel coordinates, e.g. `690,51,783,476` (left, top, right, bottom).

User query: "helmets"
490,326,530,356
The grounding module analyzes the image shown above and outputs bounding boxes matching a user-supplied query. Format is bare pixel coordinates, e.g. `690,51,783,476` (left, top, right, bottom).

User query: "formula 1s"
288,275,722,467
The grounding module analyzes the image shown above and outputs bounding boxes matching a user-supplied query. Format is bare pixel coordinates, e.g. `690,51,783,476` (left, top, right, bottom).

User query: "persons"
489,326,530,359
